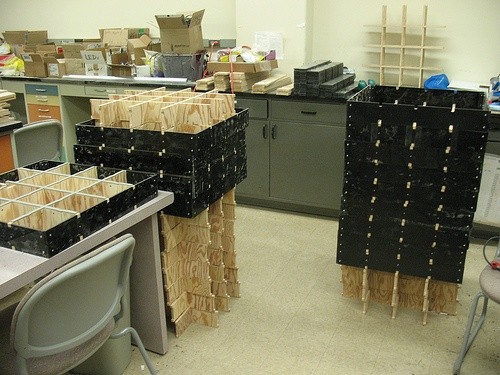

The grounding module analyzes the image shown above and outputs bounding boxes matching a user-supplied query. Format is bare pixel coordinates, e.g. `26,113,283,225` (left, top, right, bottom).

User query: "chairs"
453,238,500,375
0,233,157,375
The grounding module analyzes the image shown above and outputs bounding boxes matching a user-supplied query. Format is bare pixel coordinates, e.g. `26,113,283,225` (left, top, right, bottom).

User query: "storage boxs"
73,107,249,218
336,84,491,284
1,9,279,81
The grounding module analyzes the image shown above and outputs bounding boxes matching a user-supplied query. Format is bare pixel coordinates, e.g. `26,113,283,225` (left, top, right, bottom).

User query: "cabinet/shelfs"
234,98,346,210
23,83,91,163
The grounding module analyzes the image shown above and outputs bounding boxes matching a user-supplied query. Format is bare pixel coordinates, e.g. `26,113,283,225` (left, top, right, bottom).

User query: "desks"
0,190,175,355
